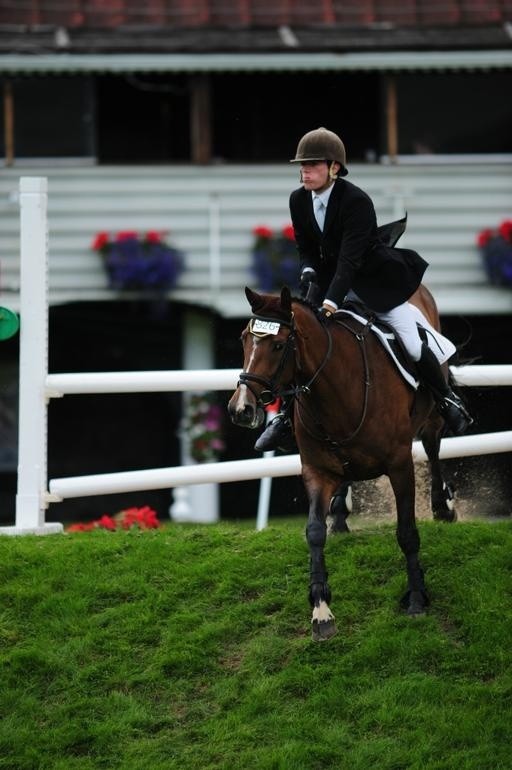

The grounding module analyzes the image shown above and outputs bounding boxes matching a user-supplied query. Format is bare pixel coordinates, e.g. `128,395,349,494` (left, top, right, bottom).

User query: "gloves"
299,271,318,293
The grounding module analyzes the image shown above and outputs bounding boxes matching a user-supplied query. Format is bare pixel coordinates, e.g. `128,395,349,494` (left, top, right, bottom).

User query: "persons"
289,126,474,437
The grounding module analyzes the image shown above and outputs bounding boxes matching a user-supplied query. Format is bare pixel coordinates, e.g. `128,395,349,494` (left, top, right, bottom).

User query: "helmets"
290,127,348,177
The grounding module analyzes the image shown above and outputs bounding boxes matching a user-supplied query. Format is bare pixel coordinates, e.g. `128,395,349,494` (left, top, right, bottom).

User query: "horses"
226,280,485,645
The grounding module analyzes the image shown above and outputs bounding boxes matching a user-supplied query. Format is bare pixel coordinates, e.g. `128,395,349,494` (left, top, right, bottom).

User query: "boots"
253,392,294,452
413,344,468,436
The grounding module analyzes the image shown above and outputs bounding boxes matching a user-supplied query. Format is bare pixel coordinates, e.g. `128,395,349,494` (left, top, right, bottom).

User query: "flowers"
91,228,163,255
176,394,224,465
255,223,295,244
478,218,511,253
